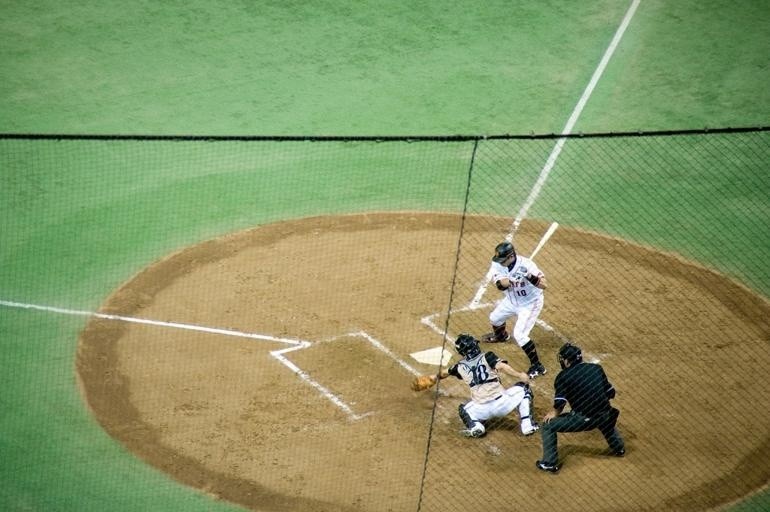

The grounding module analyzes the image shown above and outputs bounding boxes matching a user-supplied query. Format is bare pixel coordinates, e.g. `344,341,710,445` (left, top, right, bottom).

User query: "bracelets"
436,371,447,380
528,275,541,287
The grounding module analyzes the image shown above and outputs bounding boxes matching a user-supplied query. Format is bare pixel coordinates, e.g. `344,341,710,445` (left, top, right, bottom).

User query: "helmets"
492,242,513,262
455,335,480,361
557,343,582,369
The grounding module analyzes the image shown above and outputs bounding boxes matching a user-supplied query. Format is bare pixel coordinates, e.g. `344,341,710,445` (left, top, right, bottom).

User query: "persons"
535,341,626,473
411,331,540,440
481,241,549,378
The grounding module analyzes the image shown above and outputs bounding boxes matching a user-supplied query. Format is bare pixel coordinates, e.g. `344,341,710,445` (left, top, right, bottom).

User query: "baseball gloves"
409,375,434,392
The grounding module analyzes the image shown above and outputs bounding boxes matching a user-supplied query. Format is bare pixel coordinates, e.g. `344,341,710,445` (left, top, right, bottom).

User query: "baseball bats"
508,223,557,286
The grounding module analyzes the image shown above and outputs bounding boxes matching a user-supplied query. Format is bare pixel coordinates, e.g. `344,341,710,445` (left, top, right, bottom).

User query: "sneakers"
481,332,510,343
536,461,559,472
458,425,485,438
527,364,546,379
521,423,539,436
